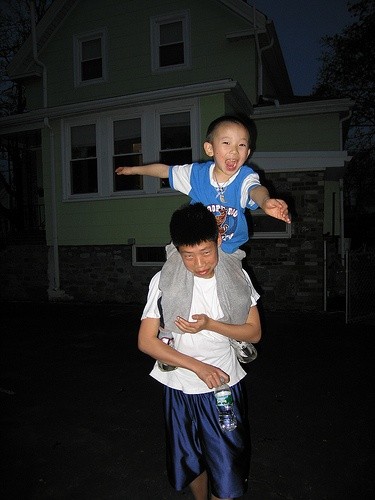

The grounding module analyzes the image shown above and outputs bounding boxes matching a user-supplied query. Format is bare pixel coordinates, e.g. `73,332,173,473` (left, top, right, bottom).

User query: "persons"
139,203,262,500
114,115,291,372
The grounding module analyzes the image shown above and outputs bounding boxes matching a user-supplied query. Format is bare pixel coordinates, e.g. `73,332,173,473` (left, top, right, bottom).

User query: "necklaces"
215,172,234,203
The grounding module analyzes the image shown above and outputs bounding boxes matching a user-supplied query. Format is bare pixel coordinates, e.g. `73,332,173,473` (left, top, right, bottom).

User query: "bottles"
157,330,178,372
214,382,238,432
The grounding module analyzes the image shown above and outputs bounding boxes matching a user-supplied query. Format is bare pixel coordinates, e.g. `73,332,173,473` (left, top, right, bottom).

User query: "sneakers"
157,336,178,372
229,337,257,363
